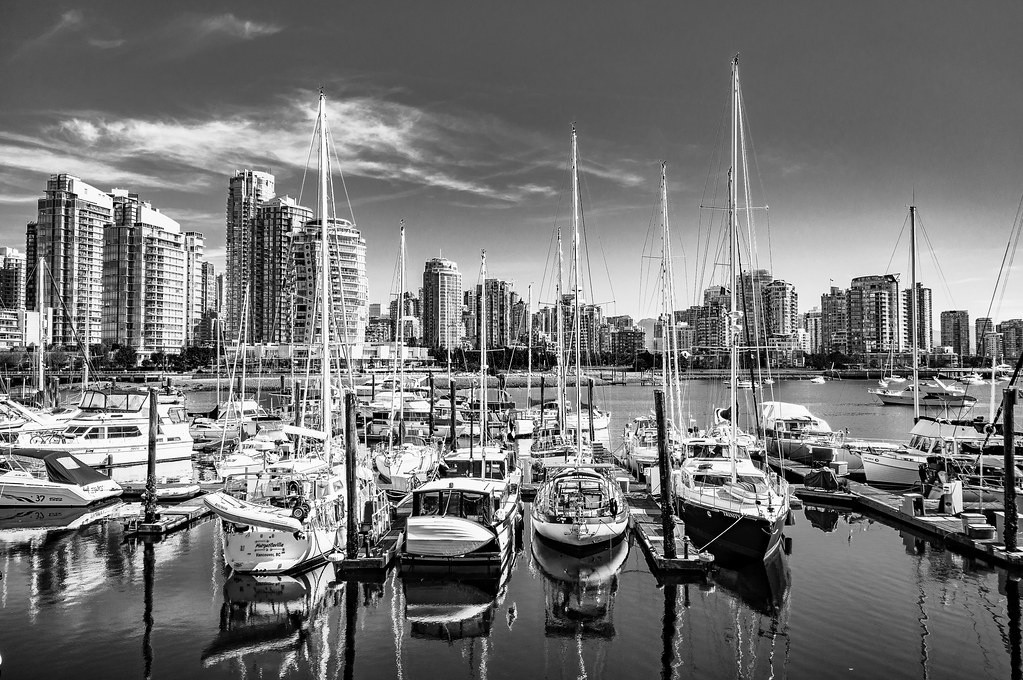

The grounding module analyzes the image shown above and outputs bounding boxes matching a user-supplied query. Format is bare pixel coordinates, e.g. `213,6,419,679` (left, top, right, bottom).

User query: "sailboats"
198,551,1023,680
2,53,1023,588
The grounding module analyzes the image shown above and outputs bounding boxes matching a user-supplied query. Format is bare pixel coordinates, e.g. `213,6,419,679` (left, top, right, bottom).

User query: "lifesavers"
697,463,713,469
983,423,996,435
